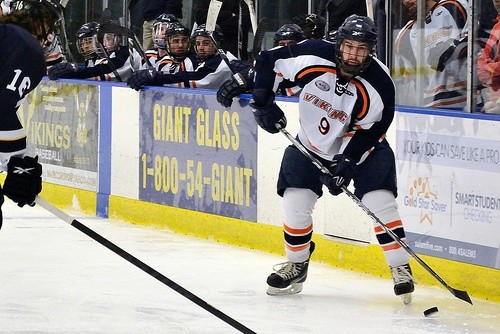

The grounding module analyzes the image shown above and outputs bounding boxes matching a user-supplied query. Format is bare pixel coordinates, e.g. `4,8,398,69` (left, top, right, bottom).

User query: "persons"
253,13,415,305
0,0,500,232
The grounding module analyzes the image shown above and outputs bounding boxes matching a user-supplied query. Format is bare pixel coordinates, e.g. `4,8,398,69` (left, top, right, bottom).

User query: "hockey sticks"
275,122,475,307
205,0,250,93
252,17,267,61
59,0,76,63
96,7,164,86
32,195,258,334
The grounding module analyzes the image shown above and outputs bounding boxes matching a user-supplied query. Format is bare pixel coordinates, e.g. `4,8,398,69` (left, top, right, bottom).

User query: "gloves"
426,37,461,73
247,100,288,135
319,154,357,195
126,68,165,92
49,61,81,81
1,155,43,208
307,13,327,41
216,71,249,107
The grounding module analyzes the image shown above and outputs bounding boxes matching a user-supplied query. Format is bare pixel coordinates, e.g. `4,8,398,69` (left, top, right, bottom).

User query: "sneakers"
264,259,309,296
389,264,417,303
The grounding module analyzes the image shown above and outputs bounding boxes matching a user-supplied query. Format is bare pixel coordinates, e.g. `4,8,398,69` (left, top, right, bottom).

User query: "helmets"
191,24,222,59
335,14,378,74
151,12,181,50
9,0,65,58
274,24,306,45
165,22,193,60
77,21,122,59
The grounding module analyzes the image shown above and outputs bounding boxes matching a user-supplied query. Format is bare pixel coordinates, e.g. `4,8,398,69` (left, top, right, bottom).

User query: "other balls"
423,306,438,316
